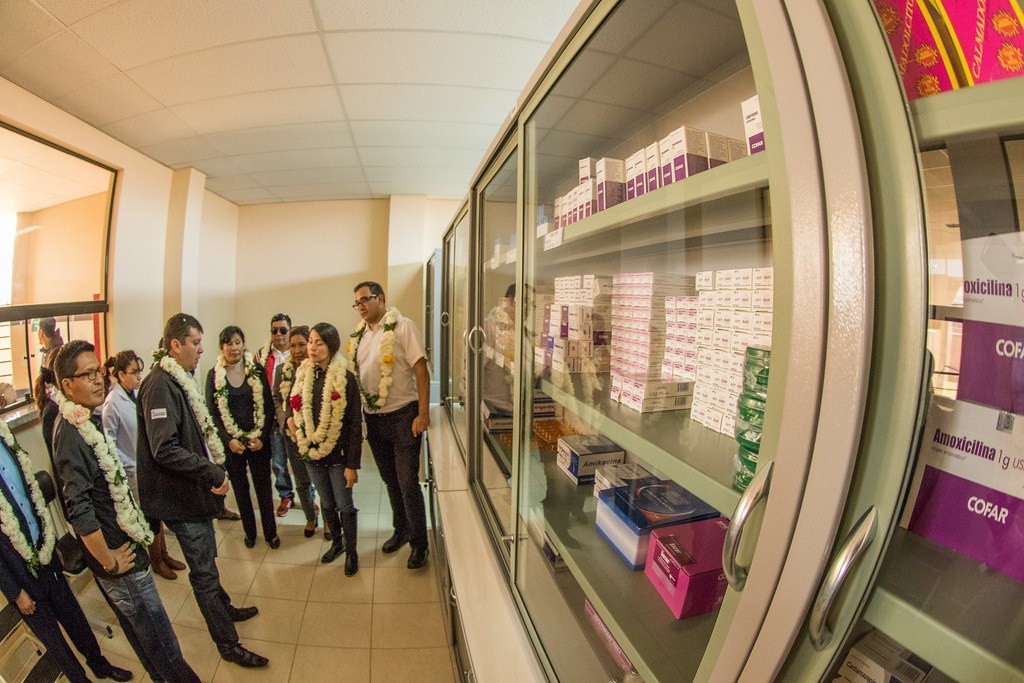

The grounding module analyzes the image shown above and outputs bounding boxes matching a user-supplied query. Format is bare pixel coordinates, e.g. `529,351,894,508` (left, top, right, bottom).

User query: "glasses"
271,327,290,335
352,295,378,312
65,367,107,380
524,297,537,309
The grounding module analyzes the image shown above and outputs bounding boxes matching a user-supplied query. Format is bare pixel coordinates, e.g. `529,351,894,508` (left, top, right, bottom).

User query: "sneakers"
312,501,320,515
276,497,295,517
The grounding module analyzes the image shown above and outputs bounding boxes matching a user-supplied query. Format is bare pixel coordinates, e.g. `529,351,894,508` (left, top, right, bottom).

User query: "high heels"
322,521,332,541
304,517,319,537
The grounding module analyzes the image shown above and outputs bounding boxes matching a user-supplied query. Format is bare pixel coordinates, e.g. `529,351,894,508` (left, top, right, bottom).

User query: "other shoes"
244,532,257,548
217,509,241,520
267,535,280,548
321,543,347,563
345,550,358,576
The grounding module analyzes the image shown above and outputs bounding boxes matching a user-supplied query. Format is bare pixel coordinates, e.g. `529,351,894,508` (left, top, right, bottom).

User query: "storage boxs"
470,1,1024,682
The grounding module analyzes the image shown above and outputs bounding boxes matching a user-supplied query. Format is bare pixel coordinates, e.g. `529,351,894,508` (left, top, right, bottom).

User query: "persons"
252,313,319,538
157,337,241,519
34,316,115,522
347,281,430,568
136,314,269,668
205,326,280,550
0,420,133,683
283,323,363,577
480,282,609,548
0,382,17,408
101,349,187,580
47,340,202,683
272,325,333,542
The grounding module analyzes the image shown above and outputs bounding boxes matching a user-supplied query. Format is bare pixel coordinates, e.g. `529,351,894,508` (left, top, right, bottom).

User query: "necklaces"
348,306,398,410
260,338,346,460
160,355,226,464
58,399,155,545
0,421,56,564
214,352,264,440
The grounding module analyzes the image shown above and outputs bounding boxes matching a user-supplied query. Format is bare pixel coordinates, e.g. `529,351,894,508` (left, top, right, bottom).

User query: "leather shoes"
382,530,411,552
407,547,430,568
228,606,259,622
221,645,268,668
86,657,133,682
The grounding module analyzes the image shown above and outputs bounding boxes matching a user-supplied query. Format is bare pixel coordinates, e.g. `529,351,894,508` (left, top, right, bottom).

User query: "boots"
158,523,187,570
147,536,177,580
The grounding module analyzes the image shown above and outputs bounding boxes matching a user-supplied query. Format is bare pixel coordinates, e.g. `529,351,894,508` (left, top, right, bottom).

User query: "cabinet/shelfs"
485,76,1023,682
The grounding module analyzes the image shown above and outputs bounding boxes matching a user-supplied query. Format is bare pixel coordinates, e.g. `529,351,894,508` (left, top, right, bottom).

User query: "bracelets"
103,558,119,574
